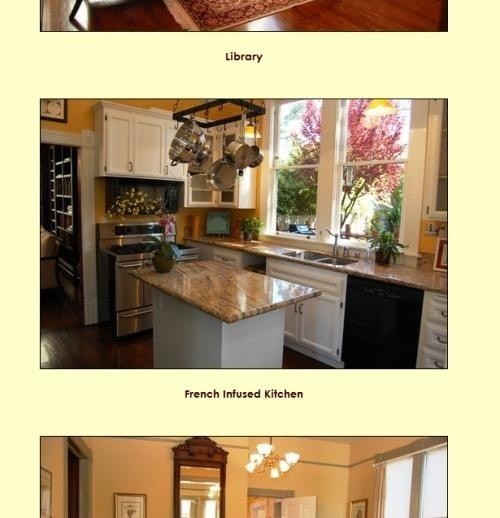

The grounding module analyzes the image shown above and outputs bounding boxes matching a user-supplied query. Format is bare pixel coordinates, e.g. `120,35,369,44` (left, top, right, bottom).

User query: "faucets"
324,227,338,256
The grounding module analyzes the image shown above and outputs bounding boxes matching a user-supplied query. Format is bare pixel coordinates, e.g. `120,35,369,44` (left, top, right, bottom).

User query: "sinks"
283,248,327,261
316,257,359,266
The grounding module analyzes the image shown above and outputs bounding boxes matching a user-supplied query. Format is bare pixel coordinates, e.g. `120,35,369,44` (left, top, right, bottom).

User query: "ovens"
116,262,202,337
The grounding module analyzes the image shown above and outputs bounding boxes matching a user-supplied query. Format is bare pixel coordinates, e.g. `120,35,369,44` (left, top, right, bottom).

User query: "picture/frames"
114,492,148,518
433,237,447,272
41,99,69,123
348,497,368,518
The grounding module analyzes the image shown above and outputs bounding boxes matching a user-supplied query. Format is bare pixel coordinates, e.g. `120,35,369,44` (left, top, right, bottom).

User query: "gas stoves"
109,241,194,261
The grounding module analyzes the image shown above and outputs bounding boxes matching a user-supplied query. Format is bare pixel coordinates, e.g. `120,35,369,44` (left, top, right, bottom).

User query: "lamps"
244,436,301,479
244,99,261,139
364,99,396,116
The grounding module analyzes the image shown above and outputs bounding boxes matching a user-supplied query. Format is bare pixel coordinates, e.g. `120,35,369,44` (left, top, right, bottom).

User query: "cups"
190,215,201,236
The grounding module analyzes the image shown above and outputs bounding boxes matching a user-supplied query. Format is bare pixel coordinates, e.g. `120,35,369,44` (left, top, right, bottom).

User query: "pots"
168,120,264,192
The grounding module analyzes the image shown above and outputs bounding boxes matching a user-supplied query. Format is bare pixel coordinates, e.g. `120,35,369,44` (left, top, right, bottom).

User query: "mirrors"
172,437,230,518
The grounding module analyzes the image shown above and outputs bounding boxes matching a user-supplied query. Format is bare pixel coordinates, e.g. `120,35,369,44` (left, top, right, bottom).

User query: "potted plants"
240,216,265,241
370,231,409,265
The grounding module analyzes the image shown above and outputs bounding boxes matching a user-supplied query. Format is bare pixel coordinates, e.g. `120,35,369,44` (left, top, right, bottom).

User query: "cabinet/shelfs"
266,258,348,369
49,144,82,287
421,98,448,222
90,100,257,210
183,240,264,270
415,290,448,368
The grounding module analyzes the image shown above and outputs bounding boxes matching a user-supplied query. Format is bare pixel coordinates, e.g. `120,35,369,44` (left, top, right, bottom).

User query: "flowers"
141,213,183,261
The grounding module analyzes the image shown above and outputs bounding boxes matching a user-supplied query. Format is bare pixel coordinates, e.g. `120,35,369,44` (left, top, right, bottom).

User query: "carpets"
163,0,316,32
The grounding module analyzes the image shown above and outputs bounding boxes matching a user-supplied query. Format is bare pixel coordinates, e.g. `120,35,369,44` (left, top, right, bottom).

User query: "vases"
152,251,175,273
